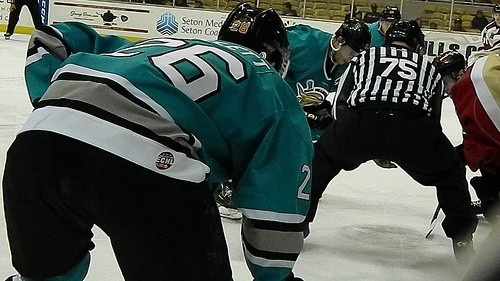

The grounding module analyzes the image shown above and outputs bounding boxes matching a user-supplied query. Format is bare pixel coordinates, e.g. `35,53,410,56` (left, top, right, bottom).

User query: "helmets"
380,5,401,23
432,51,465,77
218,3,290,78
335,20,371,53
494,4,500,12
385,19,424,52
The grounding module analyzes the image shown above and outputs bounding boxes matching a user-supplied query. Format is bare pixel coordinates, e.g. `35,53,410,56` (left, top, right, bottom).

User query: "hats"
455,18,462,23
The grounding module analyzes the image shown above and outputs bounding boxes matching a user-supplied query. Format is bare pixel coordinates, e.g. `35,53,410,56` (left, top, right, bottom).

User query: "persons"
471,10,489,30
4,0,42,40
451,18,467,32
193,0,203,8
2,2,314,281
432,51,500,224
279,2,300,19
368,5,401,47
345,3,381,24
430,21,437,29
415,18,422,28
481,5,500,51
302,18,479,264
214,18,372,223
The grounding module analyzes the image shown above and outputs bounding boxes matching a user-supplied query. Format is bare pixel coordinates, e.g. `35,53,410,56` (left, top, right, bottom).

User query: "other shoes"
453,234,474,262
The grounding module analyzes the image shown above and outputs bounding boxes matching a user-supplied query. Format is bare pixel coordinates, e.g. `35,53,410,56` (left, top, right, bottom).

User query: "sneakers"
471,200,483,216
4,33,11,39
213,180,242,219
